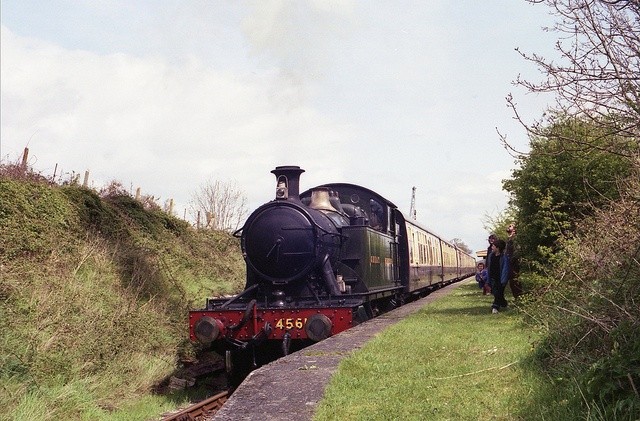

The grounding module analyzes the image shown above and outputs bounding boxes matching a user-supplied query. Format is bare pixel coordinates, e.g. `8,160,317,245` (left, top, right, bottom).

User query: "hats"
493,240,506,252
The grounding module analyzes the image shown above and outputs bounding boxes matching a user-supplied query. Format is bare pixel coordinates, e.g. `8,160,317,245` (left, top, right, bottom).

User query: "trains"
188,165,477,401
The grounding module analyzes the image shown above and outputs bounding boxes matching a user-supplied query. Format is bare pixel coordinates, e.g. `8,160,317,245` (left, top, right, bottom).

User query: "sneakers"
492,309,498,315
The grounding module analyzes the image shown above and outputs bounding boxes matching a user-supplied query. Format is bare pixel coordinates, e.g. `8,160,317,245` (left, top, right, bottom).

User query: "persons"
475,263,489,294
486,235,497,268
488,240,510,313
